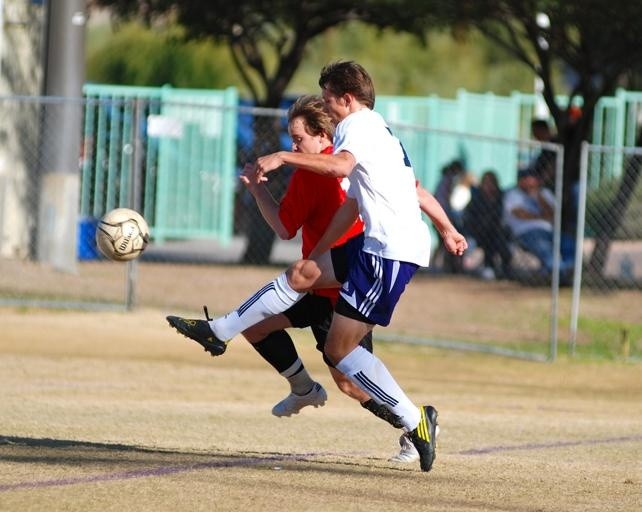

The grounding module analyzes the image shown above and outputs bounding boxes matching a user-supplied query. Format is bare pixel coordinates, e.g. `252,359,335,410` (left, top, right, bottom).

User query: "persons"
429,161,472,273
166,57,438,471
507,169,576,286
242,94,468,462
528,120,556,171
476,171,509,281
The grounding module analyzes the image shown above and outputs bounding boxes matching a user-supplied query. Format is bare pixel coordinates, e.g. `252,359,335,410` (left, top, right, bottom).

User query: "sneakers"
272,382,327,418
388,405,440,472
166,316,227,357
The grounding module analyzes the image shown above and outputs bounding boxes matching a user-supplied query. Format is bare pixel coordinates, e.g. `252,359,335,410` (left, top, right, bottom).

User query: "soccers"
93,205,151,263
